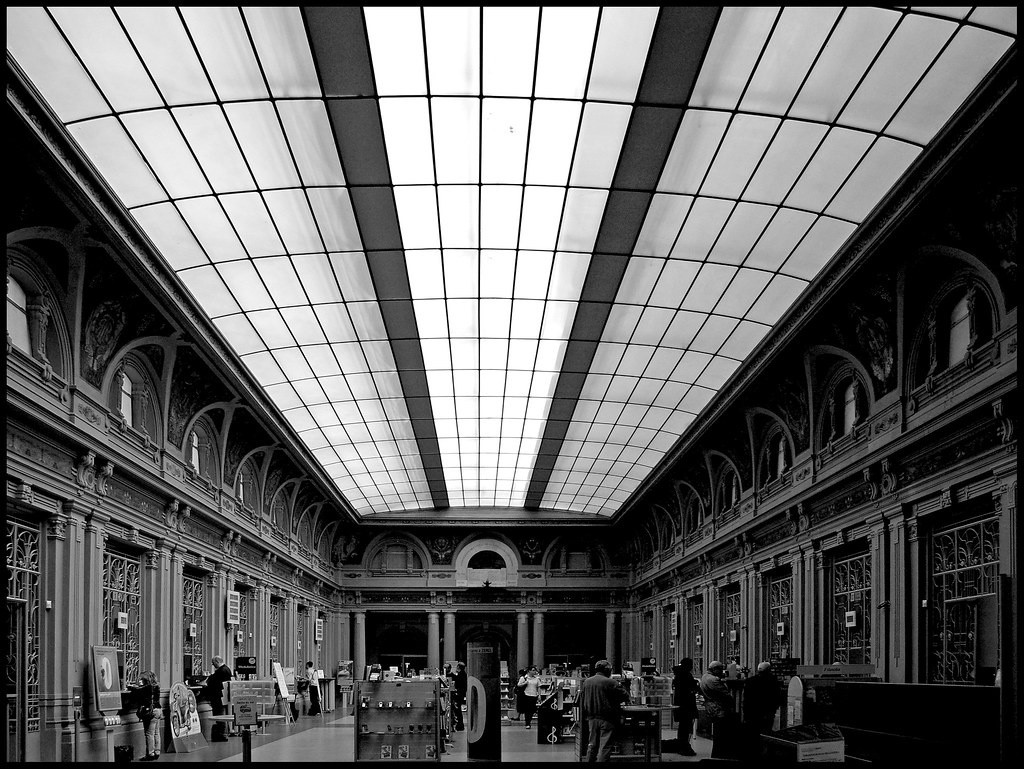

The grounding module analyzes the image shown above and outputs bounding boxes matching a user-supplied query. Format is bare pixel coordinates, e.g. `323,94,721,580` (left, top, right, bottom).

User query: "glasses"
532,670,535,671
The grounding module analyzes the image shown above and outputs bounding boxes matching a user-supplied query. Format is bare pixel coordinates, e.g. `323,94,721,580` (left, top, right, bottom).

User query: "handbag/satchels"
136,687,154,721
514,676,527,695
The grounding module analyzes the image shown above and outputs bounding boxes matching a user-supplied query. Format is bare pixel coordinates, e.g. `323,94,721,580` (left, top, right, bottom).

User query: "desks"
208,714,286,762
622,704,681,763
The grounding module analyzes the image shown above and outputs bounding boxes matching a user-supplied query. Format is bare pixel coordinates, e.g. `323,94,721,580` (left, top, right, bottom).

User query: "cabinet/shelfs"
502,674,675,734
354,681,439,762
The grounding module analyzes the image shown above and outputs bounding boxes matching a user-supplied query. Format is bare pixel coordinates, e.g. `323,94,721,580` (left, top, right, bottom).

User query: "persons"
700,661,737,758
517,667,542,730
741,662,782,734
204,654,232,742
126,670,162,762
582,660,631,762
442,662,467,731
306,661,321,717
673,657,700,757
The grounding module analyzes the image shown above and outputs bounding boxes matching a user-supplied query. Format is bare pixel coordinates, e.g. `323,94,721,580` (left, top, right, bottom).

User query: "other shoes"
526,725,531,729
139,755,159,761
